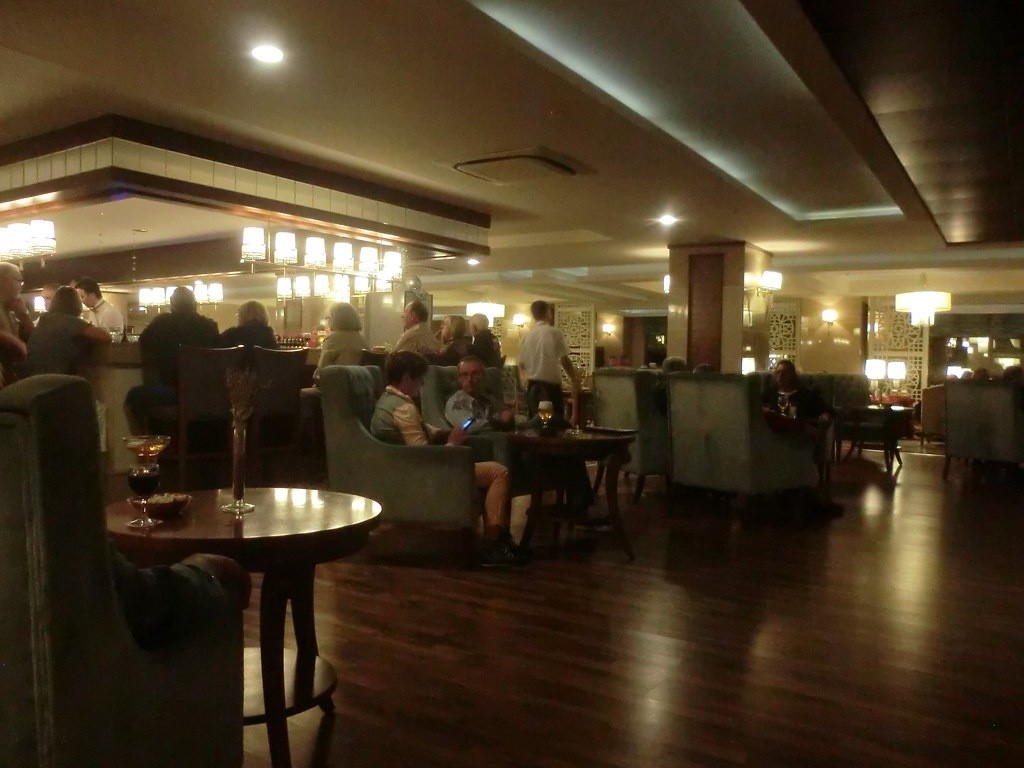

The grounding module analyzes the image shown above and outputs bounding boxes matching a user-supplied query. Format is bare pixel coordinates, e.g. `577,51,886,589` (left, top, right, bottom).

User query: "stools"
144,346,388,488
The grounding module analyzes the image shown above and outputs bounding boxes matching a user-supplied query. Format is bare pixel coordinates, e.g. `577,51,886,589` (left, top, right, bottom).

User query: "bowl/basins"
1010,338,1020,349
127,493,191,519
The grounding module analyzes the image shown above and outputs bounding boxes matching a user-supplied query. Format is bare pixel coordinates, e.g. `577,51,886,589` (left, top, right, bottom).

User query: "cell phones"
463,417,475,430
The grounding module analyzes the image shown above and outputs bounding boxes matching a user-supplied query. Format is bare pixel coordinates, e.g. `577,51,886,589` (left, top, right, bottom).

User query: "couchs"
0,365,869,768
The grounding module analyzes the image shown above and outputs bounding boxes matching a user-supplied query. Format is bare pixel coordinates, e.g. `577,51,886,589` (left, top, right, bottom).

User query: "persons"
299,302,367,421
25,287,112,375
0,261,36,340
758,359,831,452
0,331,252,651
444,355,613,531
520,302,580,423
662,357,714,373
126,287,220,491
217,300,281,352
75,276,124,341
607,355,632,368
369,351,532,569
393,302,440,365
43,281,61,313
469,315,501,367
422,317,483,367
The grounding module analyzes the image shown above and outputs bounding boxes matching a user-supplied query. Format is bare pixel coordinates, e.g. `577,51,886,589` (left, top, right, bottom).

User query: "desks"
100,487,382,768
502,431,637,562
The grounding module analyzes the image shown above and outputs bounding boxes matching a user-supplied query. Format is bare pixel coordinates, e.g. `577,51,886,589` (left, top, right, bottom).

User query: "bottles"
278,336,305,349
607,355,617,367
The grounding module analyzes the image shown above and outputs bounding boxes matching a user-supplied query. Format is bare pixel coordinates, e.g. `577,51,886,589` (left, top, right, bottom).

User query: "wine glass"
539,401,553,428
778,393,789,415
126,464,163,526
120,435,171,464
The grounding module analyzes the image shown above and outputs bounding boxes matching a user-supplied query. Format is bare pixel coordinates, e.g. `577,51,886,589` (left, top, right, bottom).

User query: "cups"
372,345,385,353
789,407,796,417
110,332,140,342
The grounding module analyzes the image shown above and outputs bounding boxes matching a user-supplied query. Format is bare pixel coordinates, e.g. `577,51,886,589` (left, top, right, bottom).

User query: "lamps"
821,308,839,325
239,227,401,299
865,361,906,391
139,281,223,307
742,271,782,326
895,273,952,327
0,219,55,260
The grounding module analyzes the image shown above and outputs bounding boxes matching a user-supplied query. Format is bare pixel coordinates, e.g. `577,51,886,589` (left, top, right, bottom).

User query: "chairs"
918,380,1024,482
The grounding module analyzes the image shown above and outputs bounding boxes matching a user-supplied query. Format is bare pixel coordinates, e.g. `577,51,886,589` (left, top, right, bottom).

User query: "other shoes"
575,515,612,533
478,540,529,570
